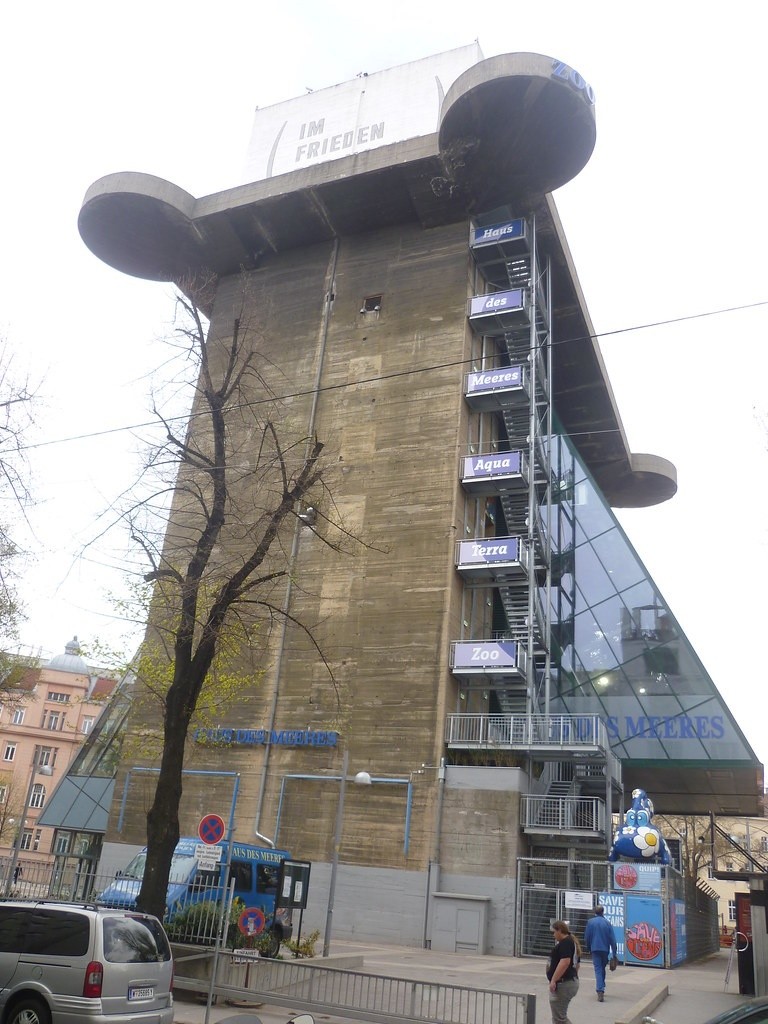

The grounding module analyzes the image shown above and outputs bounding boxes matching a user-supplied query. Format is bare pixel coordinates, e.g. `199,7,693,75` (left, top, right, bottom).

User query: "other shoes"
598,991,605,1002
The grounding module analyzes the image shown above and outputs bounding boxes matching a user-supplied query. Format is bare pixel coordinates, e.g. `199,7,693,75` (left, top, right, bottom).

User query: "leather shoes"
557,976,572,983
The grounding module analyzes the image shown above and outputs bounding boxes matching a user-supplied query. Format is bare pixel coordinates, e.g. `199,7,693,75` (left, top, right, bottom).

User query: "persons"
12,862,23,884
584,905,618,1002
545,919,583,1024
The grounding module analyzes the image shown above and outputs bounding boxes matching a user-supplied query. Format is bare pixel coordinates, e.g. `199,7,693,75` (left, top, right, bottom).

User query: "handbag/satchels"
610,956,618,970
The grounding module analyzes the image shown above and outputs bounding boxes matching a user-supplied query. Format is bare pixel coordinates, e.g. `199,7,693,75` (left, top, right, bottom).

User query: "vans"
0,897,177,1024
96,830,294,960
